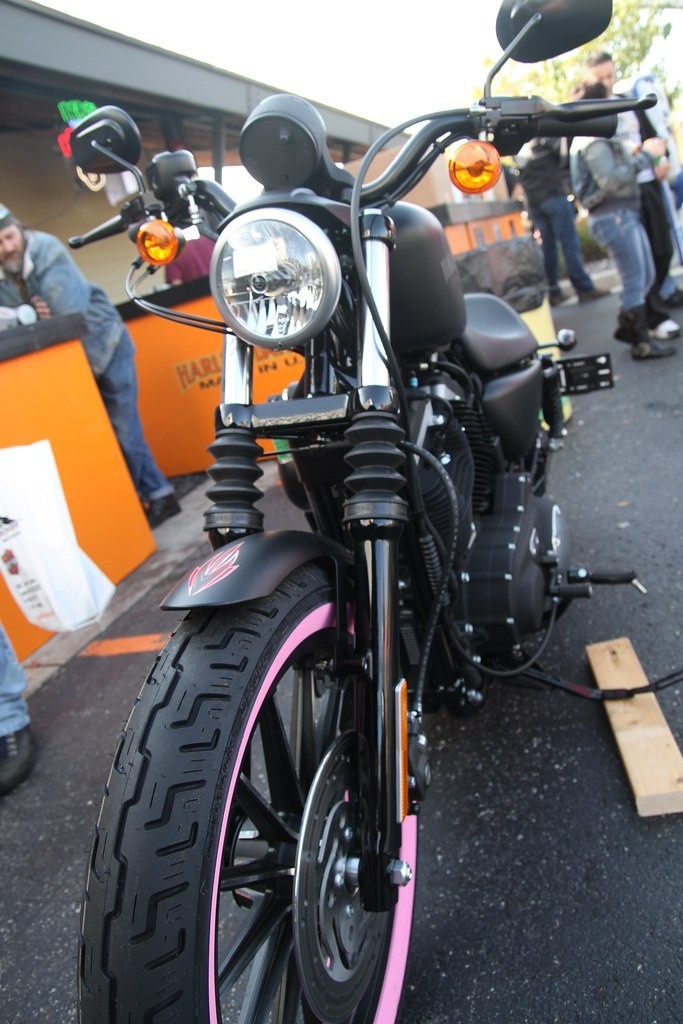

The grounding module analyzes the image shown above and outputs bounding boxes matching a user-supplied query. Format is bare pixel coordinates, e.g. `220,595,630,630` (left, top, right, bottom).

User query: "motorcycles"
54,1,659,1024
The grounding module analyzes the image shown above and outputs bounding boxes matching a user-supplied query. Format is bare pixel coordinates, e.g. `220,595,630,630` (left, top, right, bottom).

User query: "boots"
615,304,676,360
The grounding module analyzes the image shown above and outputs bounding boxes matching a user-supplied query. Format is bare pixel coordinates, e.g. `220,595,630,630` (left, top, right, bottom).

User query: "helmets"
0,200,15,228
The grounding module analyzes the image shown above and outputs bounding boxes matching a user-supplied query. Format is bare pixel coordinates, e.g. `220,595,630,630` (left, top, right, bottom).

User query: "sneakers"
648,318,680,340
0,725,36,792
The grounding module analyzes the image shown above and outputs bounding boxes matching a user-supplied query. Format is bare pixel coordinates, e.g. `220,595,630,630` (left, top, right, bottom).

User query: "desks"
113,273,308,476
0,311,159,658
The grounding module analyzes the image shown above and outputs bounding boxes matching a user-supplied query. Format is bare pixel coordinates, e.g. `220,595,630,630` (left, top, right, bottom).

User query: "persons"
567,79,675,359
514,133,613,308
164,227,218,285
658,178,683,309
0,622,37,796
0,200,180,534
578,50,680,342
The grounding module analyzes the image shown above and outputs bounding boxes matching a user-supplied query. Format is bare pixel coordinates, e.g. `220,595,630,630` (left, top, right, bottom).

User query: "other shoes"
664,290,683,307
577,290,609,302
548,291,569,306
146,494,181,529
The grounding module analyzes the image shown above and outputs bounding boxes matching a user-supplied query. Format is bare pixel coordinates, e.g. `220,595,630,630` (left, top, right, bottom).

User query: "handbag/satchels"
0,440,116,632
